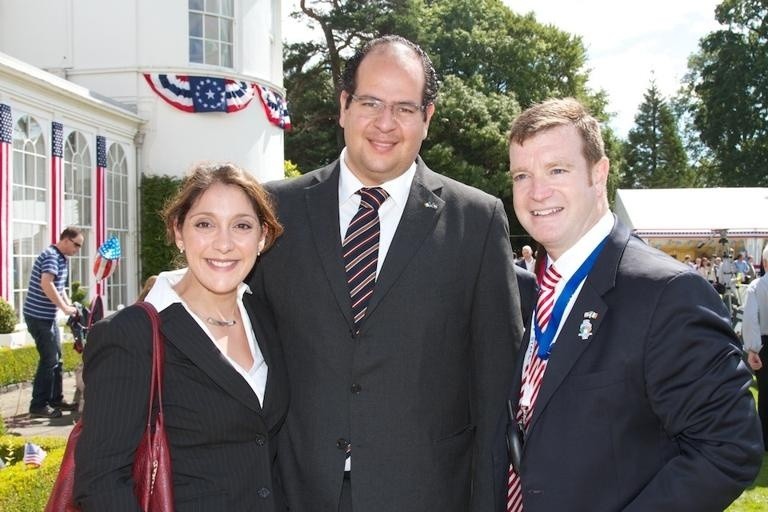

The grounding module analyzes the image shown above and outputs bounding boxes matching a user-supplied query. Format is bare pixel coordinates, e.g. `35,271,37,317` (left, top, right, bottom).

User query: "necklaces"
513,262,537,330
185,288,239,326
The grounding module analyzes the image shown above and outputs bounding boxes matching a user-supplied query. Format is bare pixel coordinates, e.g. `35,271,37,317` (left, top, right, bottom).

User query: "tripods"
716,259,742,326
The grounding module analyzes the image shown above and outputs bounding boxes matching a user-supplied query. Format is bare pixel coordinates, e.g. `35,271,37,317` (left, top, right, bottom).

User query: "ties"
502,262,563,512
339,182,394,334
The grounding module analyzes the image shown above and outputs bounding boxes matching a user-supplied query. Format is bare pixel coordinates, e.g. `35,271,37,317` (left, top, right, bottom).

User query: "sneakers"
28,404,63,418
49,397,80,411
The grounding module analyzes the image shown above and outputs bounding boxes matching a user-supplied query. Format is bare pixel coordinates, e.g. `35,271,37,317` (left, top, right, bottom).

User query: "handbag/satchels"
40,298,180,512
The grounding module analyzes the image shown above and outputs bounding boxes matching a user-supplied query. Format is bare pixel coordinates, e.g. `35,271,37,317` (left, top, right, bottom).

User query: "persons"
244,34,525,511
21,225,84,417
668,248,758,303
501,96,763,512
514,244,537,273
740,245,766,451
71,163,291,510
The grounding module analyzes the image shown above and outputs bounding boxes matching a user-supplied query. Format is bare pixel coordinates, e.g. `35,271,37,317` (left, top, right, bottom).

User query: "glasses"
67,237,83,248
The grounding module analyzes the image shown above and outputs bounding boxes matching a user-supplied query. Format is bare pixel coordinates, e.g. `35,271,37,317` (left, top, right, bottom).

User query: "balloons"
91,235,122,282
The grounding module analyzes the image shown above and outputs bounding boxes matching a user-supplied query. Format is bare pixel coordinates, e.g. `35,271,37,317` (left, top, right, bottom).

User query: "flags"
25,440,49,466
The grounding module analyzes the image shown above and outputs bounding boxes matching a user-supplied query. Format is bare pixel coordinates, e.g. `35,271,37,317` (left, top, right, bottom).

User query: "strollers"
66,294,104,410
